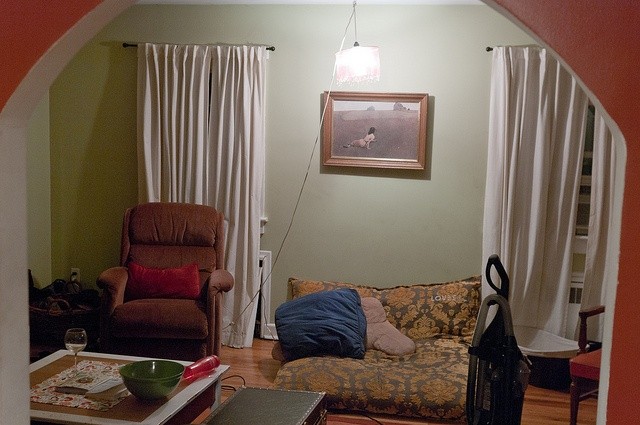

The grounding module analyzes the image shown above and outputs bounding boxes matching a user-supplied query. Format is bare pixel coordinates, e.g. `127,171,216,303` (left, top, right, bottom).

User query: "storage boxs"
512,322,589,391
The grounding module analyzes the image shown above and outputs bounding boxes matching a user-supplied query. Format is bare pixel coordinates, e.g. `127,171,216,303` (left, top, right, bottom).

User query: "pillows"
270,296,417,362
126,261,201,300
274,288,368,361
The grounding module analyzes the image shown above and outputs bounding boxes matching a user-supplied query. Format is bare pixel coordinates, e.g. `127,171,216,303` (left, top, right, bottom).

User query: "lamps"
333,2,382,85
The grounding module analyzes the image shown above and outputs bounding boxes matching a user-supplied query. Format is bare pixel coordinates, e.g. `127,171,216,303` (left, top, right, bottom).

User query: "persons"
343,127,376,150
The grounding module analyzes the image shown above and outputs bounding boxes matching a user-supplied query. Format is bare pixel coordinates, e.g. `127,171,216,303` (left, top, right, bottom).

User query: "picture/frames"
322,90,429,171
254,249,272,339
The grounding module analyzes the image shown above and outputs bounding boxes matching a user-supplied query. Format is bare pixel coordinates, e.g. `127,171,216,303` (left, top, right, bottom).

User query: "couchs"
274,275,482,424
95,202,235,360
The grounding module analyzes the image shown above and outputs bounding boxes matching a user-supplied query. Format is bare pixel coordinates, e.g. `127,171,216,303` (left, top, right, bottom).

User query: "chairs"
567,305,606,424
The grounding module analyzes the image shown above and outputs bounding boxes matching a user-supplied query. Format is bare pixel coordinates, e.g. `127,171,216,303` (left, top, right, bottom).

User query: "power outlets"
71,267,81,283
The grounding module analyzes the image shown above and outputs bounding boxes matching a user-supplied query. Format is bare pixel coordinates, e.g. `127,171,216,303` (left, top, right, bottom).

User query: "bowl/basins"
118,360,185,398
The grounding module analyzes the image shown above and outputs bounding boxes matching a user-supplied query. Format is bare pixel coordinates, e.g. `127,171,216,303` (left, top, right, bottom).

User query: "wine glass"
64,328,87,377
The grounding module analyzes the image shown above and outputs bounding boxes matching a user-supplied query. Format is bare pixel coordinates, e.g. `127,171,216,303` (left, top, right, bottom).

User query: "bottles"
183,354,220,383
